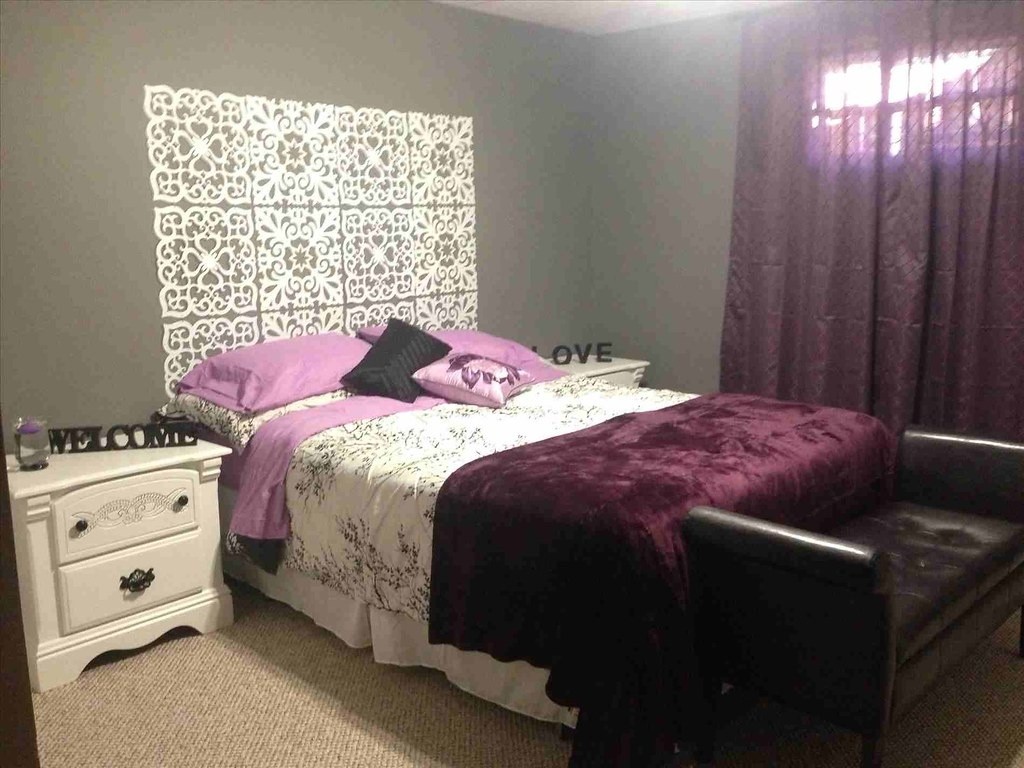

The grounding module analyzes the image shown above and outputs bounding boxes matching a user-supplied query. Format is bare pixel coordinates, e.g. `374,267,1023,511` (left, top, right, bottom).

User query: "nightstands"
545,354,650,388
6,432,234,694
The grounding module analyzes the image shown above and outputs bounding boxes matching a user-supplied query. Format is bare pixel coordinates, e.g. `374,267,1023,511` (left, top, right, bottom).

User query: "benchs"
682,431,1024,768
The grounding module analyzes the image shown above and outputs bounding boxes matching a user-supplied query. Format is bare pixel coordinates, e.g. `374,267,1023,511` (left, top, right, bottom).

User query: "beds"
157,377,899,741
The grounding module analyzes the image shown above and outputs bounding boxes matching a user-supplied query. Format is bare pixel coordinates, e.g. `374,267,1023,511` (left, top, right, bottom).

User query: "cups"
13,417,49,471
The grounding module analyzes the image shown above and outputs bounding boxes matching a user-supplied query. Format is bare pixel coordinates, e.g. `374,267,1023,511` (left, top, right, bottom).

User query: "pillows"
357,327,538,367
339,318,453,403
412,348,536,408
178,333,374,414
